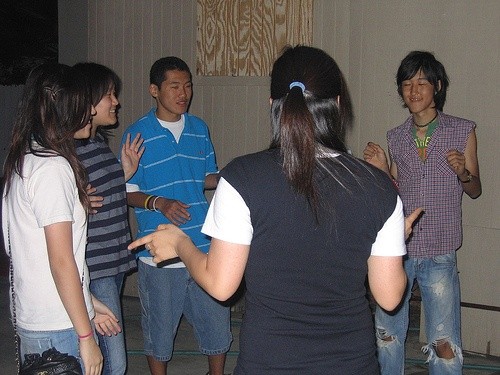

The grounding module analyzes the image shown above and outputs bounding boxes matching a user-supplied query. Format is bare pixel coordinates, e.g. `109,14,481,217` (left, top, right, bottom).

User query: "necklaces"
412,114,439,128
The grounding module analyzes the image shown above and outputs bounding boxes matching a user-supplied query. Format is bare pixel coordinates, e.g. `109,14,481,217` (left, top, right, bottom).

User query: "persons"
118,54,233,375
1,59,122,375
127,45,425,375
363,49,483,375
68,59,147,375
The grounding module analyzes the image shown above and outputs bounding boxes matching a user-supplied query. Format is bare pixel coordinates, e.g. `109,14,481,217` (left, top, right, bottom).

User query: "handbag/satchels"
19,346,83,375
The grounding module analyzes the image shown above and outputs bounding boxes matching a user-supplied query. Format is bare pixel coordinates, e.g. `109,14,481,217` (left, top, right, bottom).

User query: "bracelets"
77,330,95,339
456,170,472,183
143,194,165,212
